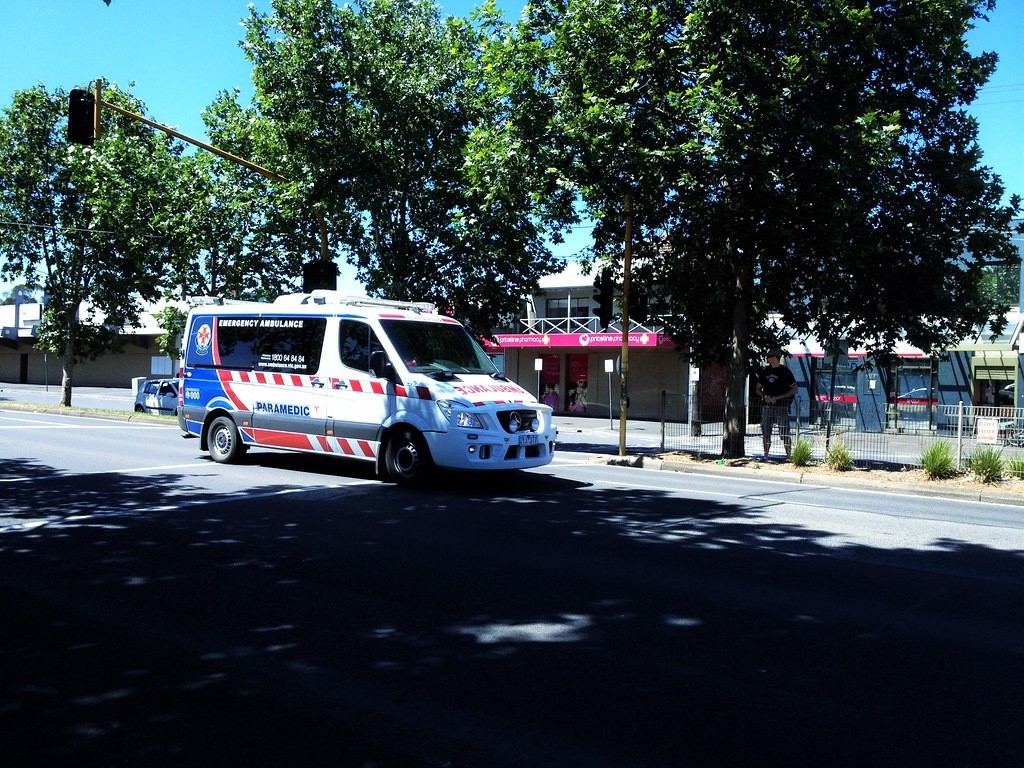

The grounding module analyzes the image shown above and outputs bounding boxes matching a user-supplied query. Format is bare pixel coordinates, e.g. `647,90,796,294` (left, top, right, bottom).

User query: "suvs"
133,379,179,415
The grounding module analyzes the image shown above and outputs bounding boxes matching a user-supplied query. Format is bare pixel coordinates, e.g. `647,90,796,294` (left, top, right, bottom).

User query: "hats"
766,348,785,358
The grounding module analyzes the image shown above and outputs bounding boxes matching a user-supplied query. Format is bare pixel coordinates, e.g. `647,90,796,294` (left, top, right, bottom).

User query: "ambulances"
177,289,559,485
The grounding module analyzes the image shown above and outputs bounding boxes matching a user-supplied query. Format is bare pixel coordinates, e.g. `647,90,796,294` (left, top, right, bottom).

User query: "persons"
755,347,799,461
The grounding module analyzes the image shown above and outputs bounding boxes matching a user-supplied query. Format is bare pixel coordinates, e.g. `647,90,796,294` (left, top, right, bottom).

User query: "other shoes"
757,455,770,462
784,456,792,463
